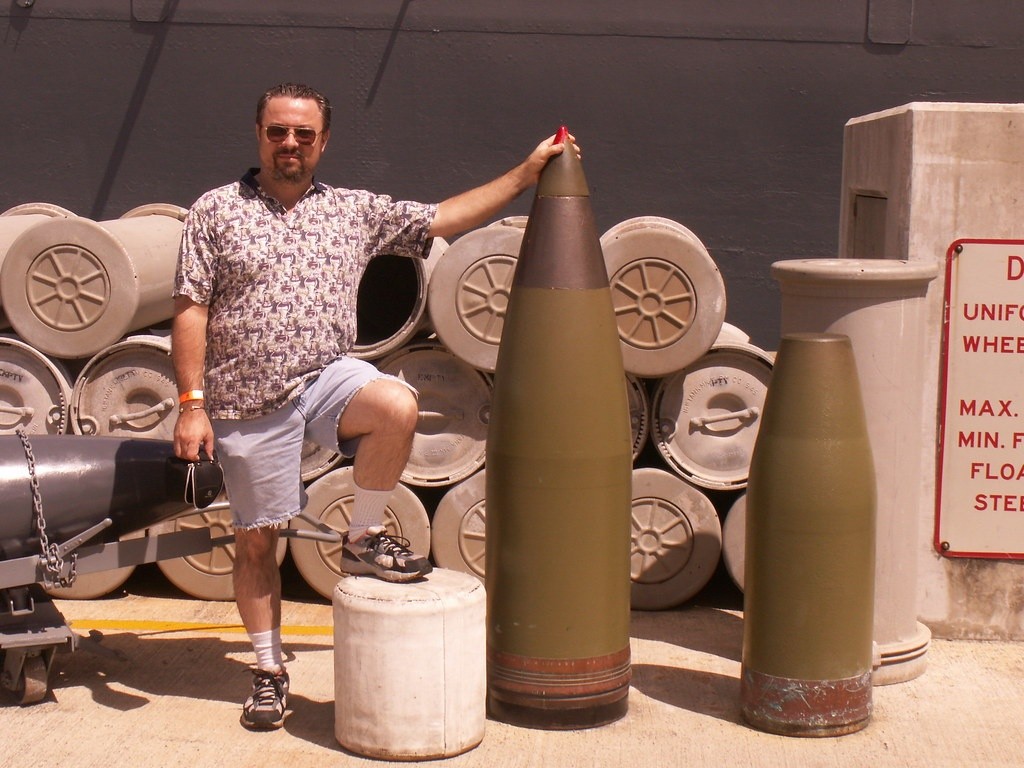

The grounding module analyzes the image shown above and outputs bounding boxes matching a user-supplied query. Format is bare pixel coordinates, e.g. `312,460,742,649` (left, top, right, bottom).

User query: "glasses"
259,124,322,145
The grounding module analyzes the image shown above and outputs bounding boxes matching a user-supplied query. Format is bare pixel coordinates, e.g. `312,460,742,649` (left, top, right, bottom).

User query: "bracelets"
178,390,206,403
176,405,204,412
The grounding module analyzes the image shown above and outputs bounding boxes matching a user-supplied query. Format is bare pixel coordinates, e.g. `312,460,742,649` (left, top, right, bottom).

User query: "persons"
168,82,584,732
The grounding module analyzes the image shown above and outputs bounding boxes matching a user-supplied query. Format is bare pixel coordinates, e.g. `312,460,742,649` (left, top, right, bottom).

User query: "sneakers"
240,665,289,732
340,524,433,583
